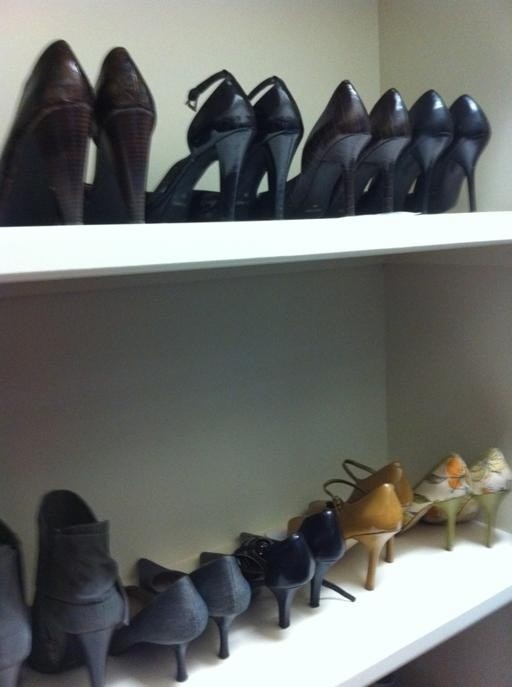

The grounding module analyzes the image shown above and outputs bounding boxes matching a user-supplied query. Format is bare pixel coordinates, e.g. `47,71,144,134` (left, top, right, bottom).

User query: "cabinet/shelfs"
0,211,512,687
